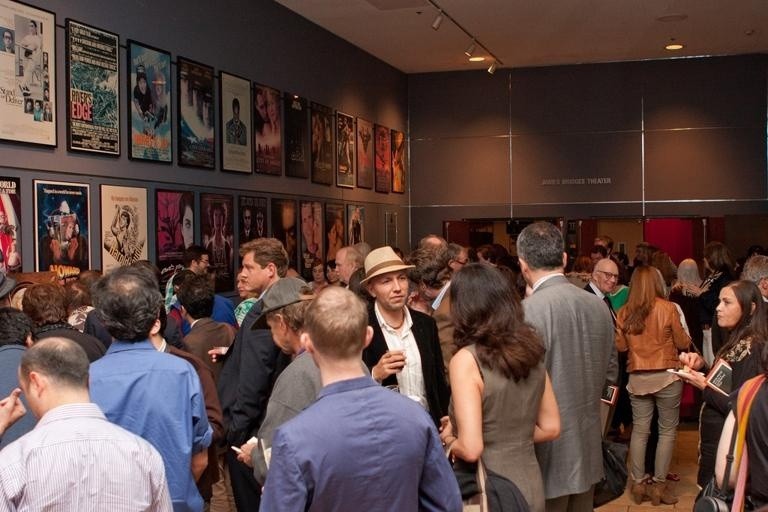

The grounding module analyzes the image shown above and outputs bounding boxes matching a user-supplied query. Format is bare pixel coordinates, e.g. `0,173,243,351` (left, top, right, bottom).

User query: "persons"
1,8,406,270
1,221,768,512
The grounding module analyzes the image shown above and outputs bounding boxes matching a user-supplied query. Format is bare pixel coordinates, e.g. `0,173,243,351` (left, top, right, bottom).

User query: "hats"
360,246,417,287
0,272,16,298
250,278,315,329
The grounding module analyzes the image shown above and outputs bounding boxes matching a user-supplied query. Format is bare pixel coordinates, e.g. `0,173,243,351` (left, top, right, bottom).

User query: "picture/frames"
325,201,346,273
357,115,375,191
270,198,299,271
253,79,284,178
2,1,62,151
336,110,356,189
1,175,24,281
199,191,236,295
236,194,269,273
176,53,219,172
284,89,311,180
301,199,325,274
153,186,197,269
30,176,94,275
126,36,176,166
312,98,335,186
392,128,406,194
63,14,125,158
347,203,366,248
98,182,151,276
219,68,253,175
375,121,392,195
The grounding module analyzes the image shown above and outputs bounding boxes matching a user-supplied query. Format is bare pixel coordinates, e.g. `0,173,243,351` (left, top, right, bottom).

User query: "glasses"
455,258,470,267
596,270,619,281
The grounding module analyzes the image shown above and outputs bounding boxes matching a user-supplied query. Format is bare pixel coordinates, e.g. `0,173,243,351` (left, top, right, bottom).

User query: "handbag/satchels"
446,441,533,512
593,440,628,509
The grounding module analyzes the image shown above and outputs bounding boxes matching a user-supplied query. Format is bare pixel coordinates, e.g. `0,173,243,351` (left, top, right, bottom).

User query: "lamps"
427,0,505,75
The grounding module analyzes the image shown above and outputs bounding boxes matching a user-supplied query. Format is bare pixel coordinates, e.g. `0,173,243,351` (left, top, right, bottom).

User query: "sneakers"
641,473,656,483
666,472,680,482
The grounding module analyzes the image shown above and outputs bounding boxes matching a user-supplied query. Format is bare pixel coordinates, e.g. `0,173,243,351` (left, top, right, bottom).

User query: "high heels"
632,483,651,504
651,483,677,505
